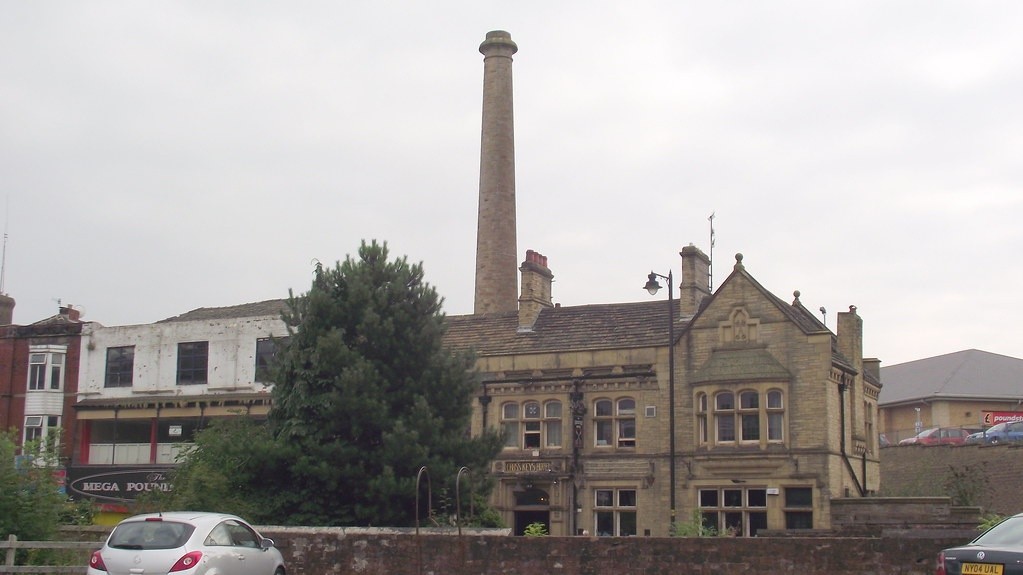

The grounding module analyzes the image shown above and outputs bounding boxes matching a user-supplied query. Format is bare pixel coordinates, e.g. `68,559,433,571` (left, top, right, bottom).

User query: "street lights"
641,270,677,537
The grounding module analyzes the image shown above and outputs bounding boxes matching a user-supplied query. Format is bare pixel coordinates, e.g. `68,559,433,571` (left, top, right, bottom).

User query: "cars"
965,421,1023,448
87,511,287,575
898,427,969,446
937,511,1023,575
